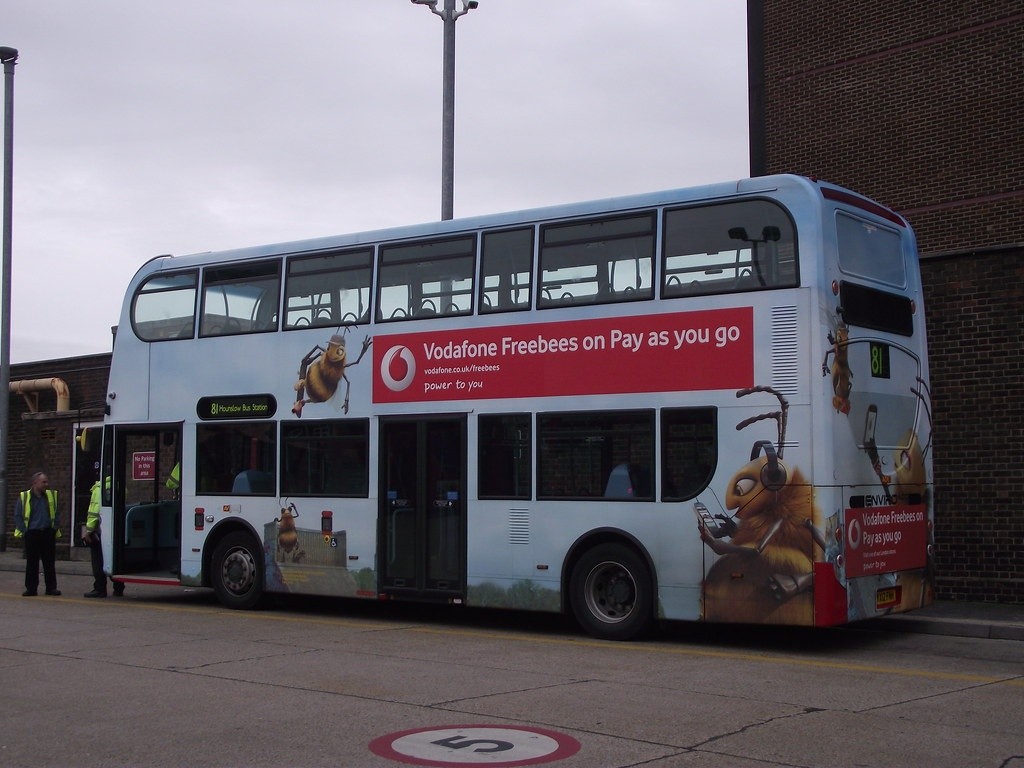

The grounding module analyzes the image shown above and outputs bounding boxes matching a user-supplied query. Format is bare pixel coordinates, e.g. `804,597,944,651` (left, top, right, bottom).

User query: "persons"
84,457,128,598
14,471,62,597
165,435,273,494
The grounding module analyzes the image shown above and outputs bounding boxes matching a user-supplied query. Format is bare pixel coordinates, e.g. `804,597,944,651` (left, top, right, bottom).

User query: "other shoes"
23,591,37,596
112,590,124,596
84,589,107,598
45,589,60,596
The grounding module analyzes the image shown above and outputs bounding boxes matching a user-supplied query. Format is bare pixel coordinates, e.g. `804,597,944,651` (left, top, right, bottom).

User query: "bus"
75,175,939,642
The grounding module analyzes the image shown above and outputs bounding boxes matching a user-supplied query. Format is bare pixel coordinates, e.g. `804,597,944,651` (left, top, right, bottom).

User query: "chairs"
232,469,274,494
209,325,222,334
250,321,266,331
624,286,637,298
560,292,575,304
294,317,310,328
687,279,703,294
340,312,358,324
264,314,277,331
482,294,491,310
664,276,683,296
390,308,407,319
444,303,460,315
221,318,242,334
542,288,552,301
359,307,383,322
178,322,193,338
734,268,754,287
414,299,436,316
312,309,334,325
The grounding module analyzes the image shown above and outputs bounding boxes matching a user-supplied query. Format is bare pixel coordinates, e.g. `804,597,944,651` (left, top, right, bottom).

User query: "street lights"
410,0,479,315
